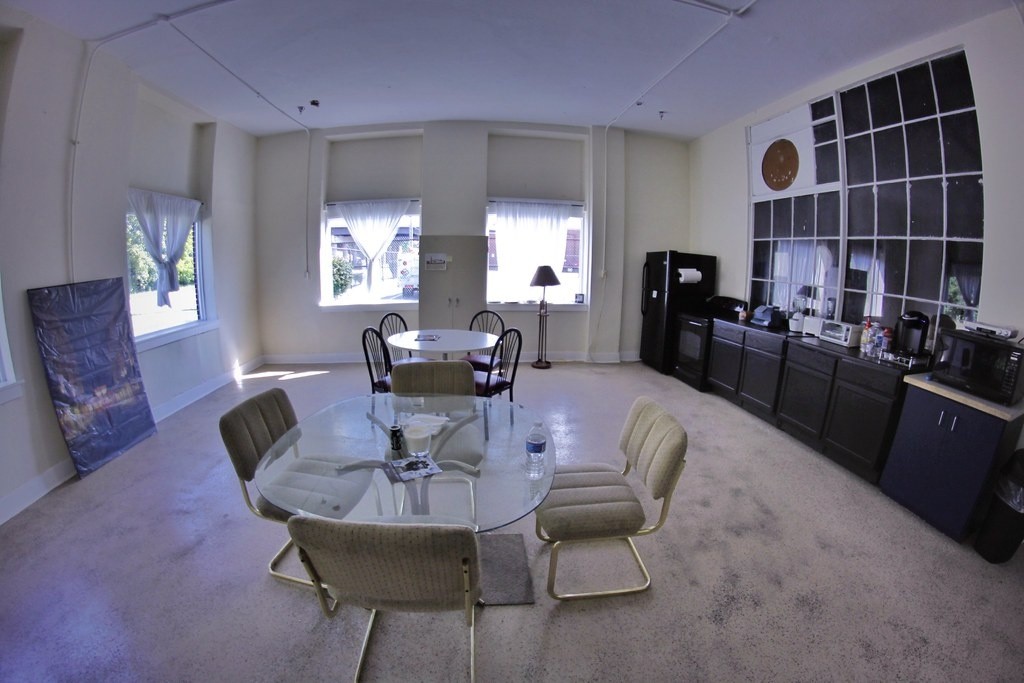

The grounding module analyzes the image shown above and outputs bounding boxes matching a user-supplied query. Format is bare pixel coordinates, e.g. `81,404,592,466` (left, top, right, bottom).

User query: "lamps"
529,266,561,369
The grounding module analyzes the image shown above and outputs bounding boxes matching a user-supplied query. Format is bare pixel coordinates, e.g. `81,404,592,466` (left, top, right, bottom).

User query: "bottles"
526,421,546,481
859,316,872,353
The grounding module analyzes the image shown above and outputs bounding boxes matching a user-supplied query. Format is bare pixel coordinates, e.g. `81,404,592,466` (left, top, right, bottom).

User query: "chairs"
384,360,482,524
219,389,383,617
287,515,481,683
534,396,687,601
362,309,522,440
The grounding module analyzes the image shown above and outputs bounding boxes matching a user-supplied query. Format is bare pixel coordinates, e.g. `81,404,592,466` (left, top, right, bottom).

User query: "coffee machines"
890,310,930,359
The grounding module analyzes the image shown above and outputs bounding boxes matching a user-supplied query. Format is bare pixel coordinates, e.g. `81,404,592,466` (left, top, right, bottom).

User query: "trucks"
396,244,419,297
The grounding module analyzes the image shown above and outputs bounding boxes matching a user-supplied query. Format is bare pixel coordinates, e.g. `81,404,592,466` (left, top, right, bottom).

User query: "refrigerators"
639,250,717,376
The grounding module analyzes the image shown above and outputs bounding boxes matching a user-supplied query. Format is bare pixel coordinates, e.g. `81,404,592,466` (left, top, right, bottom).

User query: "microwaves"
819,318,864,347
925,326,1024,408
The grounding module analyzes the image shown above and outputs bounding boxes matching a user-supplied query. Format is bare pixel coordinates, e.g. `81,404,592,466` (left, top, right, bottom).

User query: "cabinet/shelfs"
674,313,1022,544
419,235,488,360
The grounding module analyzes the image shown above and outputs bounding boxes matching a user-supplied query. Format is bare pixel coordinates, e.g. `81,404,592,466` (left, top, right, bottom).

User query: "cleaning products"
859,316,893,357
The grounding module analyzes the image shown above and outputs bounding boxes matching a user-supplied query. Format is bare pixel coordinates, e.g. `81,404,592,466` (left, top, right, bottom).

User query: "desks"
387,329,503,360
255,393,556,605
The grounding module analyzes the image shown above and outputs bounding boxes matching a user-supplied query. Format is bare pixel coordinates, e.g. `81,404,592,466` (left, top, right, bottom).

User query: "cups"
402,422,431,457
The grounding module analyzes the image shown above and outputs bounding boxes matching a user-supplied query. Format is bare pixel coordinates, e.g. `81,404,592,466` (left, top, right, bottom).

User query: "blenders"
789,296,807,332
